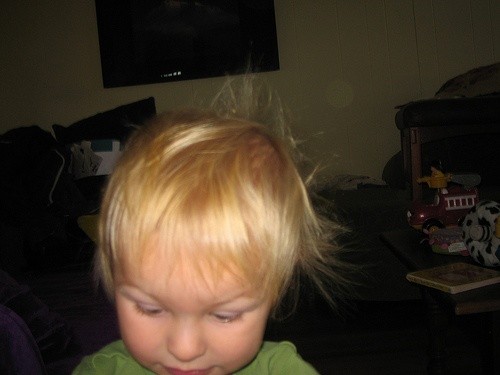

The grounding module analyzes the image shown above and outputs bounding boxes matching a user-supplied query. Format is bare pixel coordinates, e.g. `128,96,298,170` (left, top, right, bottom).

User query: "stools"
395,94,500,211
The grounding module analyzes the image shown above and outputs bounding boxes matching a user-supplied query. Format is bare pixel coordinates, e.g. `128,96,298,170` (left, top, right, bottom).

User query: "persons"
71,74,353,375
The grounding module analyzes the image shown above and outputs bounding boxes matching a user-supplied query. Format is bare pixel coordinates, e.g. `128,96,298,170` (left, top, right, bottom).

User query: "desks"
378,229,500,375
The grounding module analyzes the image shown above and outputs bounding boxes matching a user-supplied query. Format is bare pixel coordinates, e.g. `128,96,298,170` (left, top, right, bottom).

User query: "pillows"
435,62,500,98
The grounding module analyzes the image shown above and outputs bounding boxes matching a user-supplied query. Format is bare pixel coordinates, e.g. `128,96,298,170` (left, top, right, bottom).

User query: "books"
406,262,500,294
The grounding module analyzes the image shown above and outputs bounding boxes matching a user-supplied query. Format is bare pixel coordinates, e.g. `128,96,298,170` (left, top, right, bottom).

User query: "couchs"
306,186,500,330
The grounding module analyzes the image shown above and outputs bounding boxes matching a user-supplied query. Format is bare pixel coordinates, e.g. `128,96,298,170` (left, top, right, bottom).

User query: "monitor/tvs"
95,0,279,89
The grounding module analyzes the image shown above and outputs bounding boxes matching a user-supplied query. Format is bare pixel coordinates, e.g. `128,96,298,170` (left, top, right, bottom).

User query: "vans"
407,188,478,238
